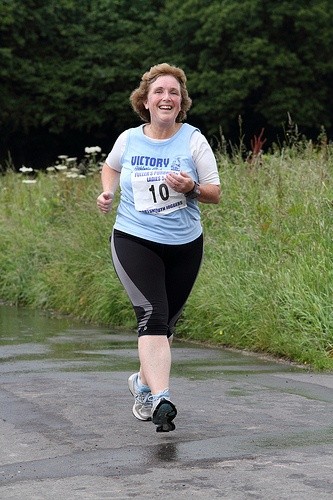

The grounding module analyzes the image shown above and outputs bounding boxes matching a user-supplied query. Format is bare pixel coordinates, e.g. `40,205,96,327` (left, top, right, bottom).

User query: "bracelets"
184,181,200,200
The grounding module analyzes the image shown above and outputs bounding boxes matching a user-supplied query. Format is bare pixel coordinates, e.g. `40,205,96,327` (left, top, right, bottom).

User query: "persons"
97,63,221,433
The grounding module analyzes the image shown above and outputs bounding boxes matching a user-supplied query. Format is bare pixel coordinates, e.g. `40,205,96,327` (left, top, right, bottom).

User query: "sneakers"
148,387,177,433
127,371,153,421
